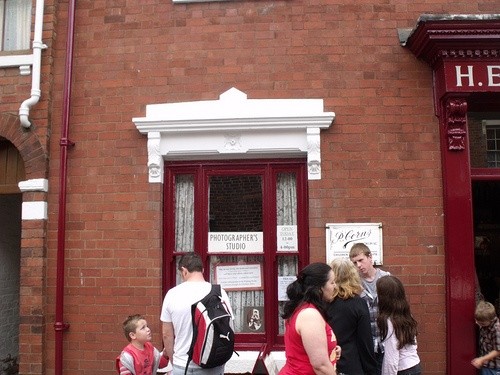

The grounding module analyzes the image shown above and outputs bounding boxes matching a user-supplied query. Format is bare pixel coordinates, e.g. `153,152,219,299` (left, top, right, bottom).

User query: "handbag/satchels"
252,342,279,375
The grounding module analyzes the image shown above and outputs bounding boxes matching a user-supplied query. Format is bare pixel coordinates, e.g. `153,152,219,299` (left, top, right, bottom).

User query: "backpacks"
184,284,239,375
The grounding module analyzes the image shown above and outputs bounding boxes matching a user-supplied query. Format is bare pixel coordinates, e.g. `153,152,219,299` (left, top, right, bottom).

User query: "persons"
115,313,170,375
160,253,235,375
276,261,341,375
327,243,421,375
471,268,500,375
